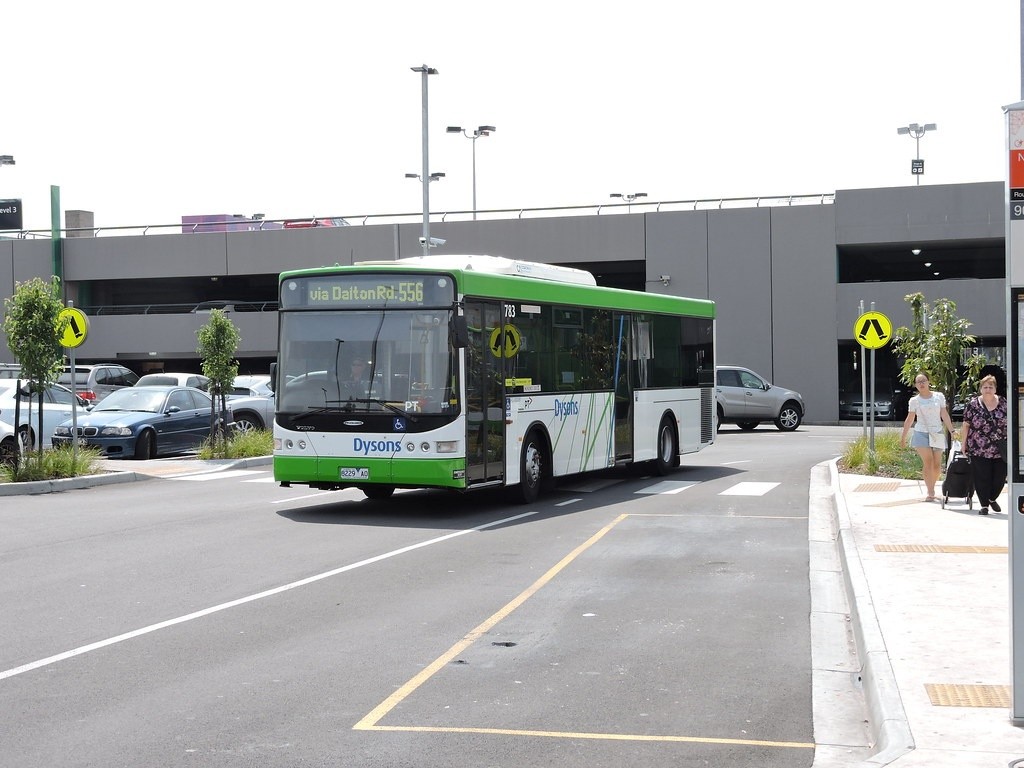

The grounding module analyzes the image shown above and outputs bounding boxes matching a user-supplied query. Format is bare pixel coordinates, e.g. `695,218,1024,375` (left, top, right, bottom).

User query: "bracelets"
951,430,955,433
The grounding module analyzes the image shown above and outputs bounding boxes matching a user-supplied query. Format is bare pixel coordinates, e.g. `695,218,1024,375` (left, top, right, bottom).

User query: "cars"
205,376,296,442
839,377,904,421
0,378,96,464
134,373,215,393
51,386,235,459
715,365,805,431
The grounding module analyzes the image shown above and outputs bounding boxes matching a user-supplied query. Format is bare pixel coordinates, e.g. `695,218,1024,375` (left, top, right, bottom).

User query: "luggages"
942,451,976,510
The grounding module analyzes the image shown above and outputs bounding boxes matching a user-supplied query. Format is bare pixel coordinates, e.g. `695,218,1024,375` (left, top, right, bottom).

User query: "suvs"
51,364,140,407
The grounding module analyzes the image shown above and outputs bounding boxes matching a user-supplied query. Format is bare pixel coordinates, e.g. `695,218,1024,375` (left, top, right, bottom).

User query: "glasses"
915,380,928,383
352,364,362,366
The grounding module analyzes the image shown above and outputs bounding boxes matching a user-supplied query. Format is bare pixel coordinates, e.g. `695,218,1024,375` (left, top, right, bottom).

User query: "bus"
266,255,719,501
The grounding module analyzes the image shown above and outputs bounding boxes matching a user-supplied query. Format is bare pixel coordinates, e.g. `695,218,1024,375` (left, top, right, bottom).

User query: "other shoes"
925,495,934,501
979,499,1001,515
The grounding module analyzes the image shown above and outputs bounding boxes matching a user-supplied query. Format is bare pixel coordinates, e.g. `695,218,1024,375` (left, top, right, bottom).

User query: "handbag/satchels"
947,440,961,470
929,431,946,450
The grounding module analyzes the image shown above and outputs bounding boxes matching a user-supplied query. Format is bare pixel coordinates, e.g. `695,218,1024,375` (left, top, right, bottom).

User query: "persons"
343,359,368,387
961,376,1007,515
901,373,958,502
